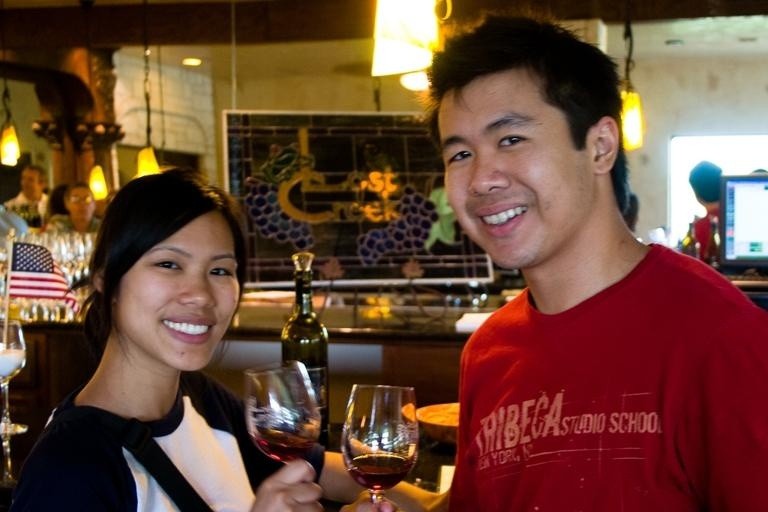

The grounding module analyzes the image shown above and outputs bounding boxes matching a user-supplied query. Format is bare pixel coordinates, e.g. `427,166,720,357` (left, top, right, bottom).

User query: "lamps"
613,0,646,152
80,30,110,202
135,45,164,177
0,82,23,170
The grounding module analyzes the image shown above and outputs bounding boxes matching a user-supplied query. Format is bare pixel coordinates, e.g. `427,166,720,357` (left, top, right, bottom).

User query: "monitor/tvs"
719,174,768,274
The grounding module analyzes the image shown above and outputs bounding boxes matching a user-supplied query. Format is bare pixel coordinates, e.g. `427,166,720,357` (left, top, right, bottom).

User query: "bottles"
0,228,96,324
280,252,333,436
704,218,723,274
682,223,699,258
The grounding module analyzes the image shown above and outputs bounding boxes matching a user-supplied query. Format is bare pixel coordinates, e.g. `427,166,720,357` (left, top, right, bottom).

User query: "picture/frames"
213,104,497,290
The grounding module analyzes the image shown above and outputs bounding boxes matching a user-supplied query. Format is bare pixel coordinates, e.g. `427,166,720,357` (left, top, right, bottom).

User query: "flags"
8,242,79,315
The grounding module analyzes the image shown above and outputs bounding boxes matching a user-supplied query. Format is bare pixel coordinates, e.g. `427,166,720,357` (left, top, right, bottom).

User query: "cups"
244,360,320,465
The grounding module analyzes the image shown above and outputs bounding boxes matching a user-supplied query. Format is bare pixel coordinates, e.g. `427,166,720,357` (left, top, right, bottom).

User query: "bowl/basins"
416,402,461,445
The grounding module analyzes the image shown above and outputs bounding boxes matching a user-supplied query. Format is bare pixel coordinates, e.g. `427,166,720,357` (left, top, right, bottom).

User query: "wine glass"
337,382,421,507
0,435,19,511
0,321,30,436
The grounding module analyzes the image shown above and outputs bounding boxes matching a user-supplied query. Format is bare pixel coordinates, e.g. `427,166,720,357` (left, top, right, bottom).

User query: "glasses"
67,193,96,205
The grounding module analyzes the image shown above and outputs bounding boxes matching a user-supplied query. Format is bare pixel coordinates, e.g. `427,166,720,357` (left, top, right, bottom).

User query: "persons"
620,162,768,274
0,166,104,240
337,15,768,512
7,166,451,512
